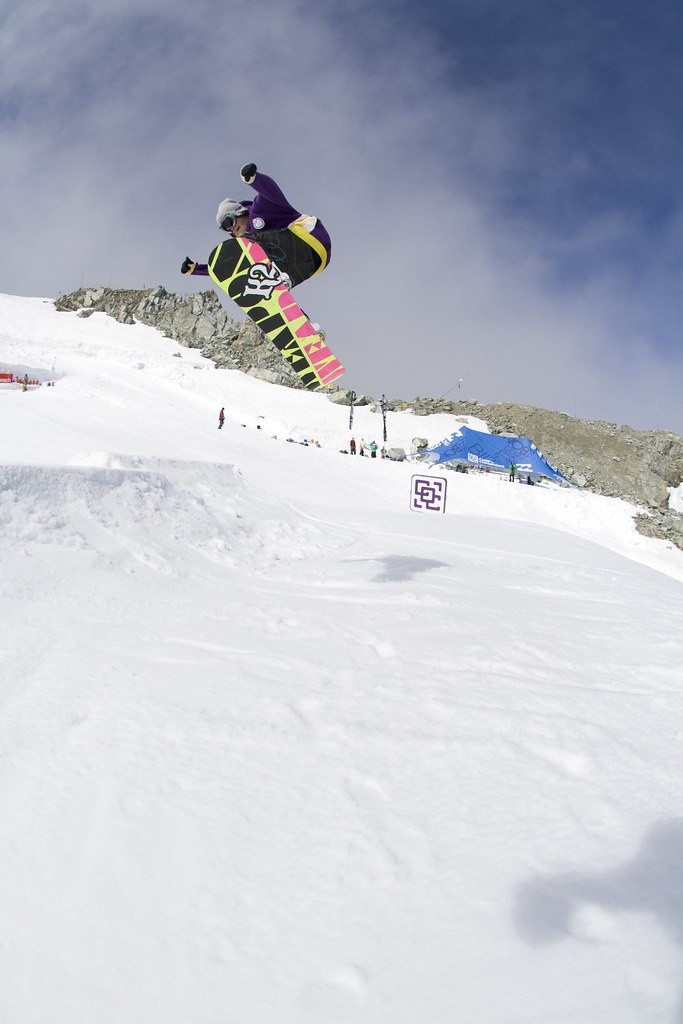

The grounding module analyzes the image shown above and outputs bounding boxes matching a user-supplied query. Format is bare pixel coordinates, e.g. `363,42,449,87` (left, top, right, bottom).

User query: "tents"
406,425,579,490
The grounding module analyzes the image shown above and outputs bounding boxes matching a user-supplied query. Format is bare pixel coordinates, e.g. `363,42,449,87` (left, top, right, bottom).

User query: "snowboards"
207,238,345,392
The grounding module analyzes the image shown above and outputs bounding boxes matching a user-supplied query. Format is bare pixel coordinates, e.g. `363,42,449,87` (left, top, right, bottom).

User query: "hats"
216,198,248,230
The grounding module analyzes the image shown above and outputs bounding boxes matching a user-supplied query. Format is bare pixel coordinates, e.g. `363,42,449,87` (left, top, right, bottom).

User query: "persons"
350,437,387,459
218,407,225,429
180,163,331,333
509,462,534,486
22,374,28,392
455,463,474,473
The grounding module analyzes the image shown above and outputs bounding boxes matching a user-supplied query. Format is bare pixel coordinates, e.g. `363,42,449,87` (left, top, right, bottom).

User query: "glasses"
220,212,236,232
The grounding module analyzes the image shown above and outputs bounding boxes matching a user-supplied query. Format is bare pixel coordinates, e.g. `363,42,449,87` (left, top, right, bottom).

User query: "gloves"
181,256,195,275
241,163,257,182
